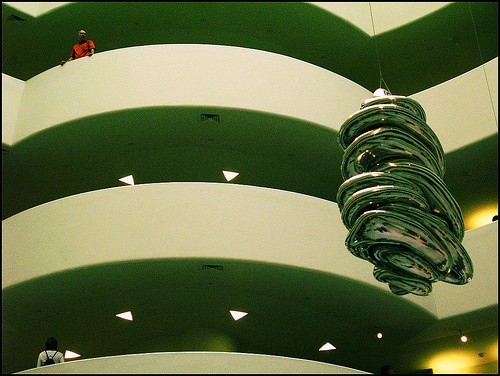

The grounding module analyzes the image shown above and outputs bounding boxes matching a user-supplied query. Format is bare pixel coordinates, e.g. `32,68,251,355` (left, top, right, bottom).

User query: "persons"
380,365,394,374
60,29,95,66
37,337,65,367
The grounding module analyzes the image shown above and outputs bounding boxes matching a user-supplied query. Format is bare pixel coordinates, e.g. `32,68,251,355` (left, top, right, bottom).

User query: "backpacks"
44,350,59,365
